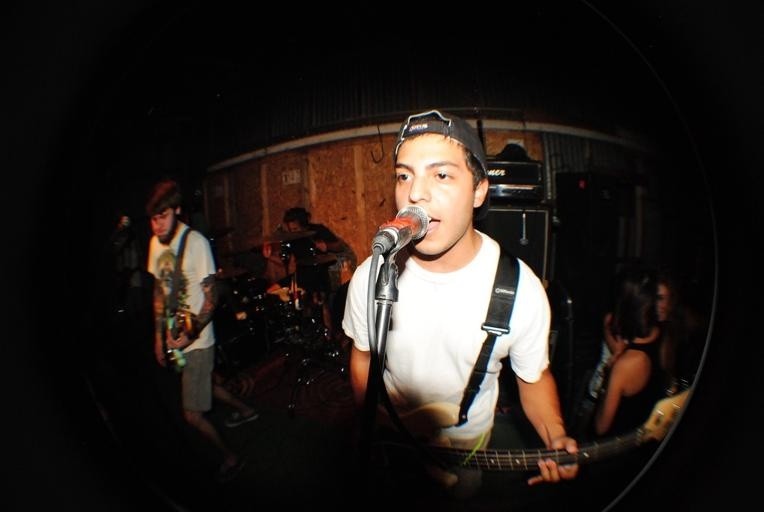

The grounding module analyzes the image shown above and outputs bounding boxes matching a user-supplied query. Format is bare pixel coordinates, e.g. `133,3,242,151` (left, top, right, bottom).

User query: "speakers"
473,209,549,283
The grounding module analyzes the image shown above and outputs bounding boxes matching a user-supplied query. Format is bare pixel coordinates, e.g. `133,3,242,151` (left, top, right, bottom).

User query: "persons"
588,244,704,442
142,181,263,486
340,109,581,510
281,207,358,332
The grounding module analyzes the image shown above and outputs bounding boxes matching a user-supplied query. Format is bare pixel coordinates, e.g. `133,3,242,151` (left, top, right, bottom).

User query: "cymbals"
214,266,250,280
259,231,315,242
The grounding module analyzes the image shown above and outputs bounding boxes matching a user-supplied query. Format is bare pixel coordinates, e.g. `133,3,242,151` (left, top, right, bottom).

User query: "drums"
210,303,258,378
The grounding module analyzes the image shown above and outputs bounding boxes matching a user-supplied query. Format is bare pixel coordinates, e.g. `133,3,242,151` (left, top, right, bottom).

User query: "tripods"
266,262,352,416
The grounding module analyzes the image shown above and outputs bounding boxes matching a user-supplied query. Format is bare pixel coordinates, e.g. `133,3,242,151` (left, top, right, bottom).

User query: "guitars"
162,313,187,367
358,393,687,509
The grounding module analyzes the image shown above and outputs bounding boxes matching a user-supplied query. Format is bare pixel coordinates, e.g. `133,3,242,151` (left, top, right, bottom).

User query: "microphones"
519,214,530,246
371,204,428,255
104,215,130,248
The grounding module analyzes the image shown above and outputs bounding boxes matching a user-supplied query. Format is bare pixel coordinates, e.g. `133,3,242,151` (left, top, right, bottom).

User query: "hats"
393,108,488,179
